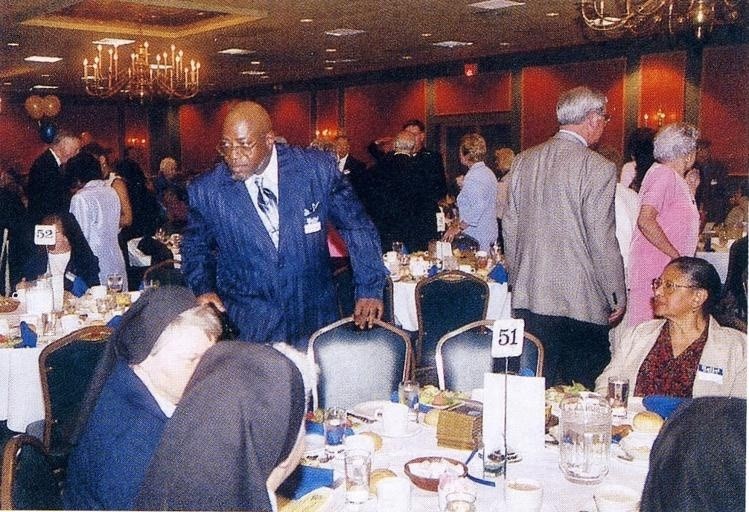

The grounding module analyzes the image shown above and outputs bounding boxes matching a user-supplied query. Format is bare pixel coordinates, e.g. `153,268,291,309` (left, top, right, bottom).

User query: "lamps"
78,30,205,105
573,2,748,45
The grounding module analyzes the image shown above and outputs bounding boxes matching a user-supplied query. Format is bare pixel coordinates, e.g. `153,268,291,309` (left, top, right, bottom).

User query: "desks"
685,234,746,284
380,250,509,333
276,384,666,512
125,234,184,270
1,289,144,435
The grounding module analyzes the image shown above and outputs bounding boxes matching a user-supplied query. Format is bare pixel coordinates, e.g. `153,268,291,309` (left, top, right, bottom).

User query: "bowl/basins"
403,455,470,491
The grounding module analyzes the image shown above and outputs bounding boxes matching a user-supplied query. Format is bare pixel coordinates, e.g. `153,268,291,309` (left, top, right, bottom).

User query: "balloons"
24,96,61,143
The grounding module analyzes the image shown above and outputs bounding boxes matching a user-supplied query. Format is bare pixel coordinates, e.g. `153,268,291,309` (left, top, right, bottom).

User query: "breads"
369,468,396,493
359,432,382,451
424,409,440,426
634,411,664,432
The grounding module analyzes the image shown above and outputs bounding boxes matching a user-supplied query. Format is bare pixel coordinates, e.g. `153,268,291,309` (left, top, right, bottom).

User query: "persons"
136,340,320,509
684,139,732,225
502,88,630,388
620,127,656,194
351,120,447,254
179,101,391,351
639,395,746,511
443,132,515,256
60,284,350,511
592,253,746,408
333,134,365,185
0,124,189,298
698,177,749,253
627,122,701,325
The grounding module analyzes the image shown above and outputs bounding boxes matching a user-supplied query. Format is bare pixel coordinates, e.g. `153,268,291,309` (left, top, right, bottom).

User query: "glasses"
605,113,611,123
652,278,697,294
216,134,263,157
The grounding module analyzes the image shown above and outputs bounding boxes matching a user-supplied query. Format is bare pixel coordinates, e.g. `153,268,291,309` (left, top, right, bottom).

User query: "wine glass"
156,226,165,241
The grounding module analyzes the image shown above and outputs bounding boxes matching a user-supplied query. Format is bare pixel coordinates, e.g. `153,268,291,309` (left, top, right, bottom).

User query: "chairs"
24,325,118,456
435,321,545,399
0,433,63,509
308,315,411,418
413,268,490,375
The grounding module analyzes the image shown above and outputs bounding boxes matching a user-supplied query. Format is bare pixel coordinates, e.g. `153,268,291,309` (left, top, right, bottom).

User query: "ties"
254,177,279,237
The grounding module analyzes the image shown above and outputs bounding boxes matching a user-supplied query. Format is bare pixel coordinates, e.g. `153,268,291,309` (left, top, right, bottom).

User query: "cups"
283,376,694,512
0,272,159,349
714,221,744,249
380,241,476,283
